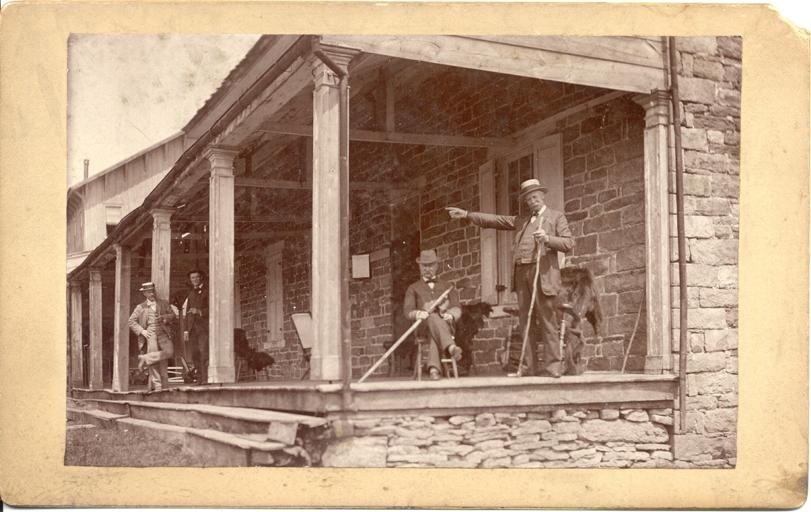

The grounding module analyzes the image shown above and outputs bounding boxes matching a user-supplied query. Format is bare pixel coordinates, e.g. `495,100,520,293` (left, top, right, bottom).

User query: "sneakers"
520,367,535,376
137,355,145,375
452,346,463,361
543,366,561,379
430,370,442,382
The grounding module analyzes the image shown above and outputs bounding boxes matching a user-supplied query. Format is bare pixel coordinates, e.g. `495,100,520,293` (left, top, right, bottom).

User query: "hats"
517,178,549,200
186,268,206,280
138,281,158,292
415,248,441,264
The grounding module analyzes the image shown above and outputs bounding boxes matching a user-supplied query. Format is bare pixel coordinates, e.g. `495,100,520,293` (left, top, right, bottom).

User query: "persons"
443,176,577,379
401,248,465,381
127,281,176,393
176,266,210,385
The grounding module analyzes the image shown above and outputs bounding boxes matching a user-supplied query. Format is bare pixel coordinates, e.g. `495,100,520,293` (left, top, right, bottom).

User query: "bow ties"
425,279,436,284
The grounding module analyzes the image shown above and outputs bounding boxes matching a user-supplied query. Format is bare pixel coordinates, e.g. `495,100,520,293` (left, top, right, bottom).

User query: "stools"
413,327,459,381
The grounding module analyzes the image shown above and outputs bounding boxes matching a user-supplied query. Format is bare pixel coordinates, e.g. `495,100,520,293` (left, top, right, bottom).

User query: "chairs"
502,266,594,378
234,327,276,381
